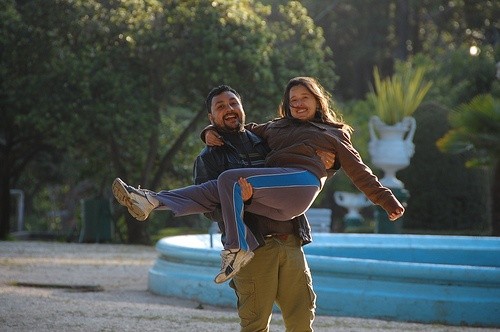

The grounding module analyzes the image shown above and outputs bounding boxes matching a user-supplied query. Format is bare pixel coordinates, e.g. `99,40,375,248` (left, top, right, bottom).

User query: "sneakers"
112,177,154,221
213,246,255,284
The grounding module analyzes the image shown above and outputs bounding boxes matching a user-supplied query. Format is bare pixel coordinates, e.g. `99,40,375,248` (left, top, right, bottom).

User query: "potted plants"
334,172,374,219
367,62,433,188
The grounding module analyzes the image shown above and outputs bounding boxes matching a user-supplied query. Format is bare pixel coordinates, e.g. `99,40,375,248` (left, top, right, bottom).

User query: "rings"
325,160,328,163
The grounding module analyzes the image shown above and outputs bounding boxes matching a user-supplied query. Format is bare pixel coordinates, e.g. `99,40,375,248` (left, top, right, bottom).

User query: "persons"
194,80,316,332
111,76,406,284
